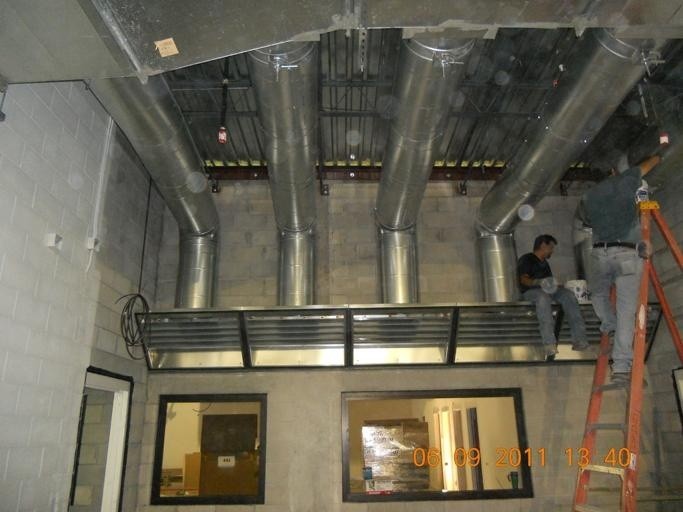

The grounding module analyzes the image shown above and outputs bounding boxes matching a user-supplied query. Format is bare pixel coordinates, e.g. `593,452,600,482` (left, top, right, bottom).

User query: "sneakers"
610,371,648,389
607,330,616,345
544,343,559,356
572,340,596,352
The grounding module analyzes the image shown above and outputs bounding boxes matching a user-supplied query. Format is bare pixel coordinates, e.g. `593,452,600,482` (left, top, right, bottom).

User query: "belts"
592,242,639,248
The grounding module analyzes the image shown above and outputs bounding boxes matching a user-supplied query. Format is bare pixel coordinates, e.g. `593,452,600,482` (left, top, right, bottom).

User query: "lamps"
646,80,669,144
218,57,229,143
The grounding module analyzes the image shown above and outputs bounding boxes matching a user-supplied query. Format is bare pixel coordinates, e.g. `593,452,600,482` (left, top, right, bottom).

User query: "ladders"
572,202,683,512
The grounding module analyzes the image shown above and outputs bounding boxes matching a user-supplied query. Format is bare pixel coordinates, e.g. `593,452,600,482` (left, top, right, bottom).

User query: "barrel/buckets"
564,279,588,304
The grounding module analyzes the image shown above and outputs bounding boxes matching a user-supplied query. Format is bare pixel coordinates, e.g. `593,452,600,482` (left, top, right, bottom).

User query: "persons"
572,155,659,390
517,233,596,356
